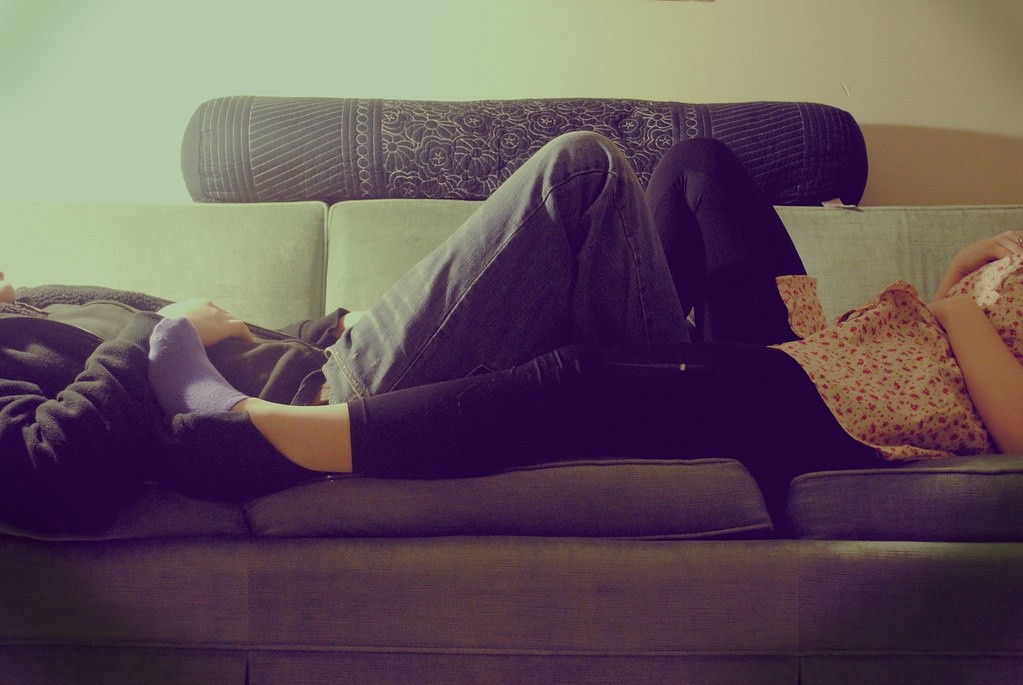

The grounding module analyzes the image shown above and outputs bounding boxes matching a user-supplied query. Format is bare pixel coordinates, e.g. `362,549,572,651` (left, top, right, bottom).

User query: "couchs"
0,199,1023,685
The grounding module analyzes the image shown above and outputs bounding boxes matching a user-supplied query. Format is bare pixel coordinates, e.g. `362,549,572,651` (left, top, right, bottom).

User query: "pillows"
181,98,868,206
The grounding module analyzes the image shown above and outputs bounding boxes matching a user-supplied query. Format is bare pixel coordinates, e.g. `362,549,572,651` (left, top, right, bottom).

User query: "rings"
1019,238,1023,246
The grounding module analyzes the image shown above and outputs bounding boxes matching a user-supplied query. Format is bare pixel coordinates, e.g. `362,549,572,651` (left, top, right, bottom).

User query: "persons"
147,138,1023,511
0,129,691,536
